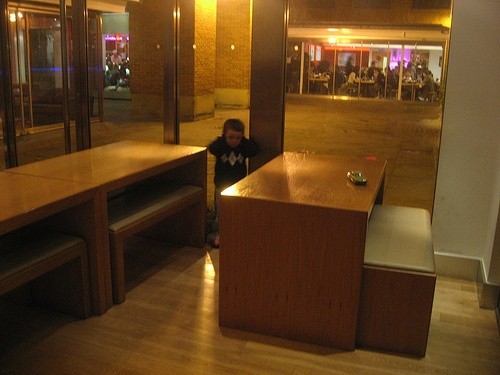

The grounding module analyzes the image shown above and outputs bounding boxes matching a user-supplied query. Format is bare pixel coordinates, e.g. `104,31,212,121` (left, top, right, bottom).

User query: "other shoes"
206,237,220,248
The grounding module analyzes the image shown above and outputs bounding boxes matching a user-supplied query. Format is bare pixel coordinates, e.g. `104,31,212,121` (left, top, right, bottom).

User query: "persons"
287,48,348,100
395,60,434,101
209,118,260,245
344,55,401,102
104,49,130,88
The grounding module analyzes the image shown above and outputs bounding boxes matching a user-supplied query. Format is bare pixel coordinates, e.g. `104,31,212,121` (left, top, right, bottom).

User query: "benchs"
106,179,206,306
0,224,96,321
424,92,437,103
356,205,437,358
346,86,358,96
391,89,404,99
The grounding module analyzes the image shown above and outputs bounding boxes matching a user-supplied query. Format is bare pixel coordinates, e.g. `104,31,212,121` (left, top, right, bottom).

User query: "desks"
0,172,106,327
403,82,422,102
311,78,330,94
355,79,375,97
218,152,387,350
4,140,207,310
